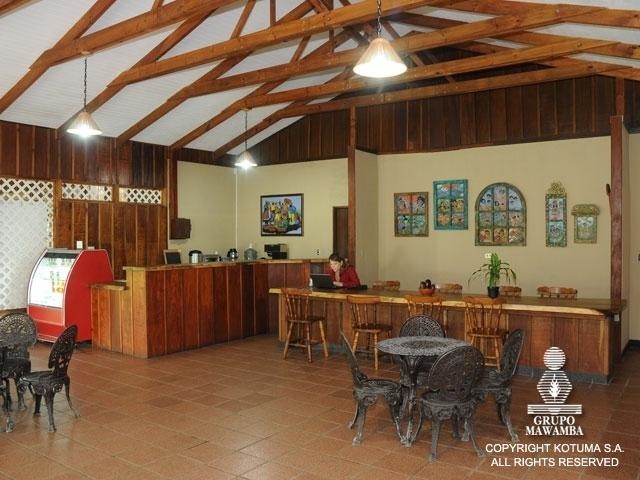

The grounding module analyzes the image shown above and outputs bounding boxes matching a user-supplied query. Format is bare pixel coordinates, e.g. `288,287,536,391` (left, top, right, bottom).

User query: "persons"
549,201,561,239
413,196,425,214
324,253,359,287
478,190,521,243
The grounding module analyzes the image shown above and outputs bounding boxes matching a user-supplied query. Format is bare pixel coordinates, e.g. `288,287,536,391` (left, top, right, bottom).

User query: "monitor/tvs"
163,249,181,265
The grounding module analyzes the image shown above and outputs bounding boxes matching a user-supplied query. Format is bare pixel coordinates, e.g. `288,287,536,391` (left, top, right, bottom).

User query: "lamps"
67,51,103,136
233,104,259,169
352,1,409,79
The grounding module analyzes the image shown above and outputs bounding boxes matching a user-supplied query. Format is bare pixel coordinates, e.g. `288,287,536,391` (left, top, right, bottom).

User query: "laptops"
311,274,341,289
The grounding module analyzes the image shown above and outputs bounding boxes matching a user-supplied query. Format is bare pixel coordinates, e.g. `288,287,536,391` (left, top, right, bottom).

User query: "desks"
268,285,629,385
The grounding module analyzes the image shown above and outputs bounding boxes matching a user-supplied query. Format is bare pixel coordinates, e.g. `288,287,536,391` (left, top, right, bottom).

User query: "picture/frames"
392,176,598,248
259,193,304,238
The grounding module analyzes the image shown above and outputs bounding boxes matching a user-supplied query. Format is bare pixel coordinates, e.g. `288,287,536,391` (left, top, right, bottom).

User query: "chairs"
0,312,81,433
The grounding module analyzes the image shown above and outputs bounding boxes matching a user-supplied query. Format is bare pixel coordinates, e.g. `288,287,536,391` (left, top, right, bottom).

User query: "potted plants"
469,253,517,299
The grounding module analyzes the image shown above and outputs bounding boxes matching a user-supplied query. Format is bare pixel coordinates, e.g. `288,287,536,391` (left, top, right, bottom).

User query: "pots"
188,249,239,263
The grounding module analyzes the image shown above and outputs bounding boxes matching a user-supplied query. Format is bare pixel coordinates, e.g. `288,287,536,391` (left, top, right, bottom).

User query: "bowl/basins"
419,288,434,297
